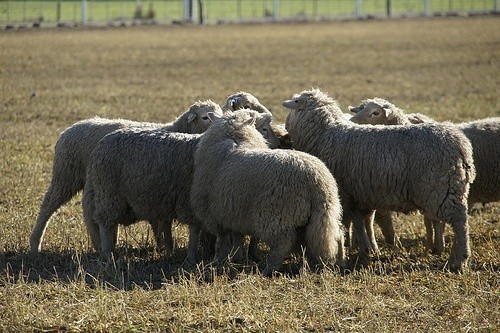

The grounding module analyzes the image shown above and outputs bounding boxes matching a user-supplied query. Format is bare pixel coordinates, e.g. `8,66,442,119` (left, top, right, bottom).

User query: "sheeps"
82,103,281,265
222,89,273,130
281,85,476,270
189,111,349,277
349,95,499,253
29,97,224,254
